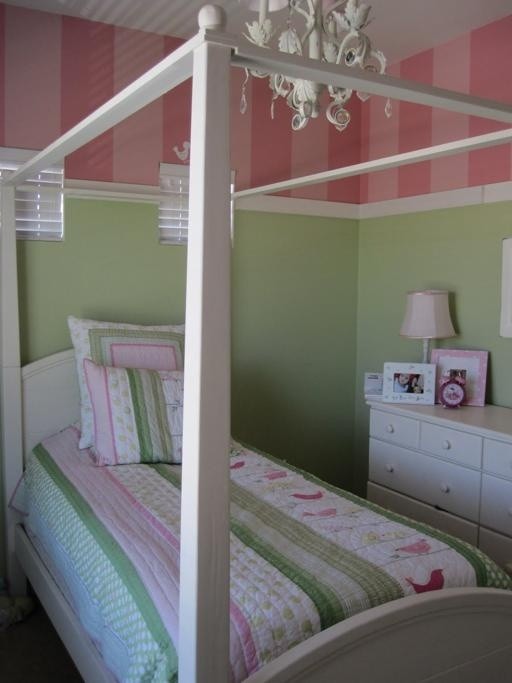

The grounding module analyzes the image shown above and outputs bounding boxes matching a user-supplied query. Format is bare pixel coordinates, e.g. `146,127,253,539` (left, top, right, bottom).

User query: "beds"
0,3,512,683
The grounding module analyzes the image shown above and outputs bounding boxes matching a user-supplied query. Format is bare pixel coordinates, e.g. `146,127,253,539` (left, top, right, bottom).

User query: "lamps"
238,0,393,129
399,289,458,365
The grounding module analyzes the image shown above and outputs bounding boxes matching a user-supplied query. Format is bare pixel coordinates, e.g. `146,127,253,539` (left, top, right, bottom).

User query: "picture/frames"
382,362,437,406
430,349,489,407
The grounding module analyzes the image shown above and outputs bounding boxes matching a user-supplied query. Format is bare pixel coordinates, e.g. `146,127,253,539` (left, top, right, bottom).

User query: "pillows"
82,356,185,467
67,314,186,451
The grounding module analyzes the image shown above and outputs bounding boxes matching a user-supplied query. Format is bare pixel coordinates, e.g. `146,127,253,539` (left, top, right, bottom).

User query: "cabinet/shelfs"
365,401,511,576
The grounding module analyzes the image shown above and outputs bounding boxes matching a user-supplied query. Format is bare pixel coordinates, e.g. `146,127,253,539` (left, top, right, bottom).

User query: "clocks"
439,374,466,409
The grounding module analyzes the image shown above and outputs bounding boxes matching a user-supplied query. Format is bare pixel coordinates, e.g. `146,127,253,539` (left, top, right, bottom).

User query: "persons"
393,373,424,393
